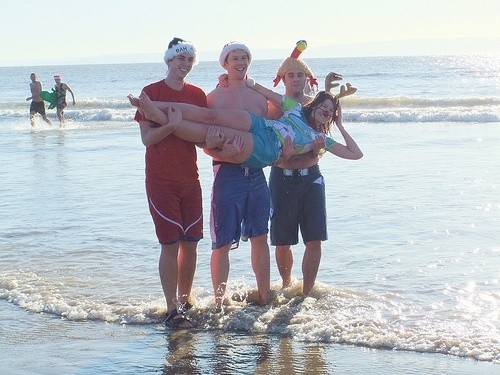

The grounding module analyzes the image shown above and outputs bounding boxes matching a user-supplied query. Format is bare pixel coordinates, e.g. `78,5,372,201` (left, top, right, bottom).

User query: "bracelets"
246,76,256,88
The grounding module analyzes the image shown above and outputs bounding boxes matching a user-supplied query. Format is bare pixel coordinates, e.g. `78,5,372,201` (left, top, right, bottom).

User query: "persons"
127,73,364,168
269,56,328,296
25,73,53,128
203,40,271,312
51,74,76,128
134,37,225,328
